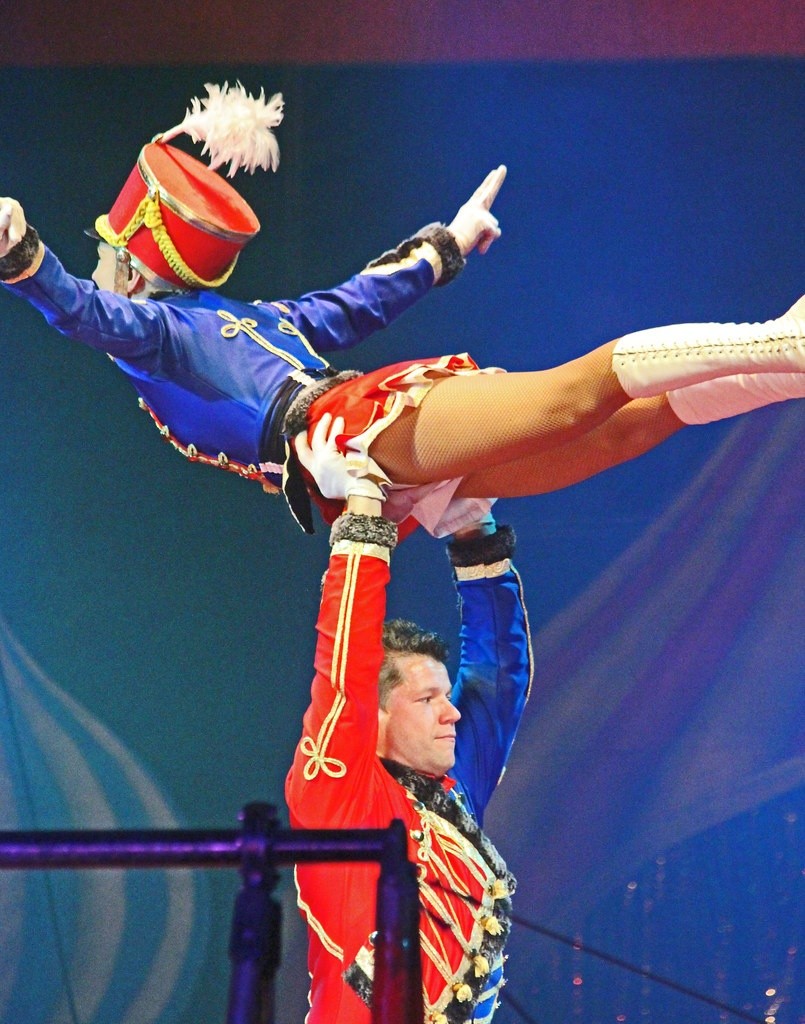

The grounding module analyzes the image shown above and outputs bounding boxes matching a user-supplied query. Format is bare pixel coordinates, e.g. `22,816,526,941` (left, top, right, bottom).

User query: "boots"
666,373,805,425
612,295,805,398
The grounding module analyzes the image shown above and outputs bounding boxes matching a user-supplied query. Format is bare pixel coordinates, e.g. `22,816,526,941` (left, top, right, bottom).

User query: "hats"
84,79,285,291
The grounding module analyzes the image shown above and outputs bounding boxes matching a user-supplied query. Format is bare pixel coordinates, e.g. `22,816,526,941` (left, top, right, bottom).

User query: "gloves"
0,195,26,259
449,164,510,257
295,412,387,502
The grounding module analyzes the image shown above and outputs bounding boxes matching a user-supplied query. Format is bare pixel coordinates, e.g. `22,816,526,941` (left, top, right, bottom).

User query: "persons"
291,409,535,1024
0,80,805,539
